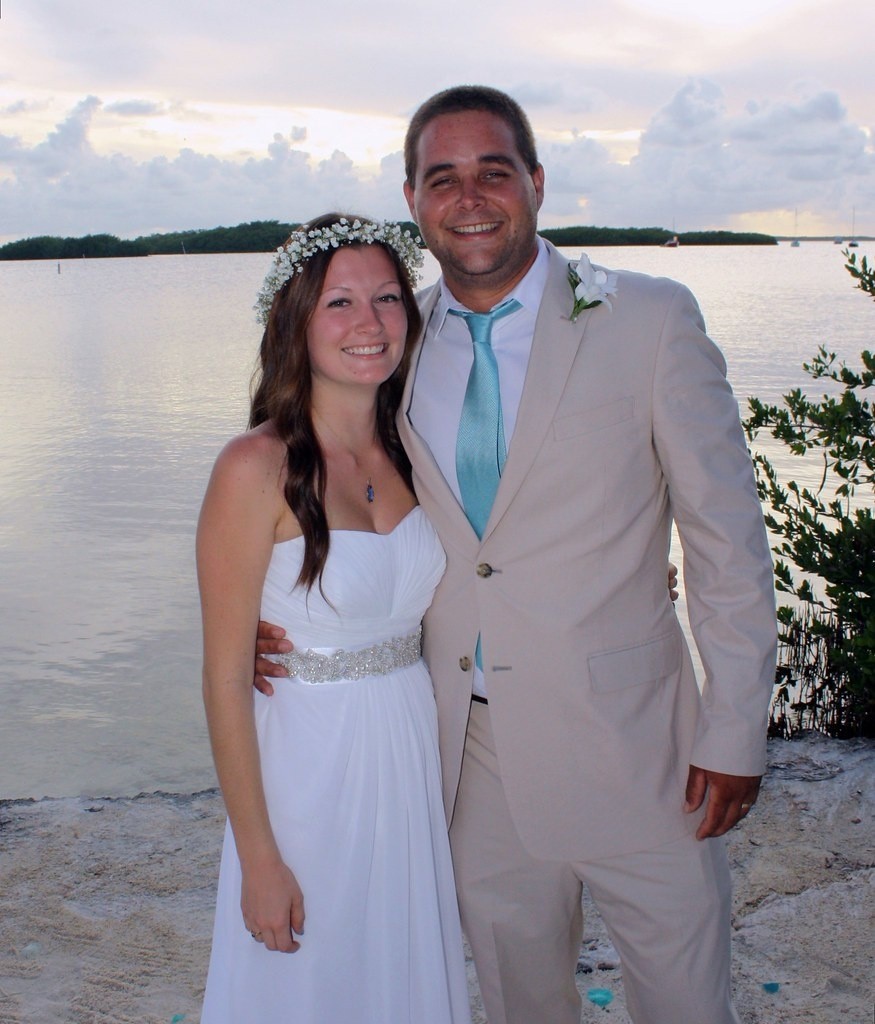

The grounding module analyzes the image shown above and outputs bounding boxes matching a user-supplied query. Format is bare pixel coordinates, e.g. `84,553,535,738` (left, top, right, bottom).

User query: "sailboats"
848,206,859,247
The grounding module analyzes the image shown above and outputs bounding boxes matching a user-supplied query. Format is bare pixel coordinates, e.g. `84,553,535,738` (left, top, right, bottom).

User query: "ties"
447,297,522,671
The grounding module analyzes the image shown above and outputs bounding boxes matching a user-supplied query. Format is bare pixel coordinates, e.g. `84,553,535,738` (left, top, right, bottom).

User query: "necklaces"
311,402,379,504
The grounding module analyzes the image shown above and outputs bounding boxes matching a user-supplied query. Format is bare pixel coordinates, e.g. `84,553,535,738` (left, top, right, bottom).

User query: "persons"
196,213,679,1024
252,86,779,1024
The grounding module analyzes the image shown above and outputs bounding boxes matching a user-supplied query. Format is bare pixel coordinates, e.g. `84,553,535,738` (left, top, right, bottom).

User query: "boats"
834,237,843,244
664,219,679,247
790,239,800,247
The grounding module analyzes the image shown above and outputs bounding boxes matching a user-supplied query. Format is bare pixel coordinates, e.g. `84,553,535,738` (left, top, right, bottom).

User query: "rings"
251,931,261,937
742,803,753,808
